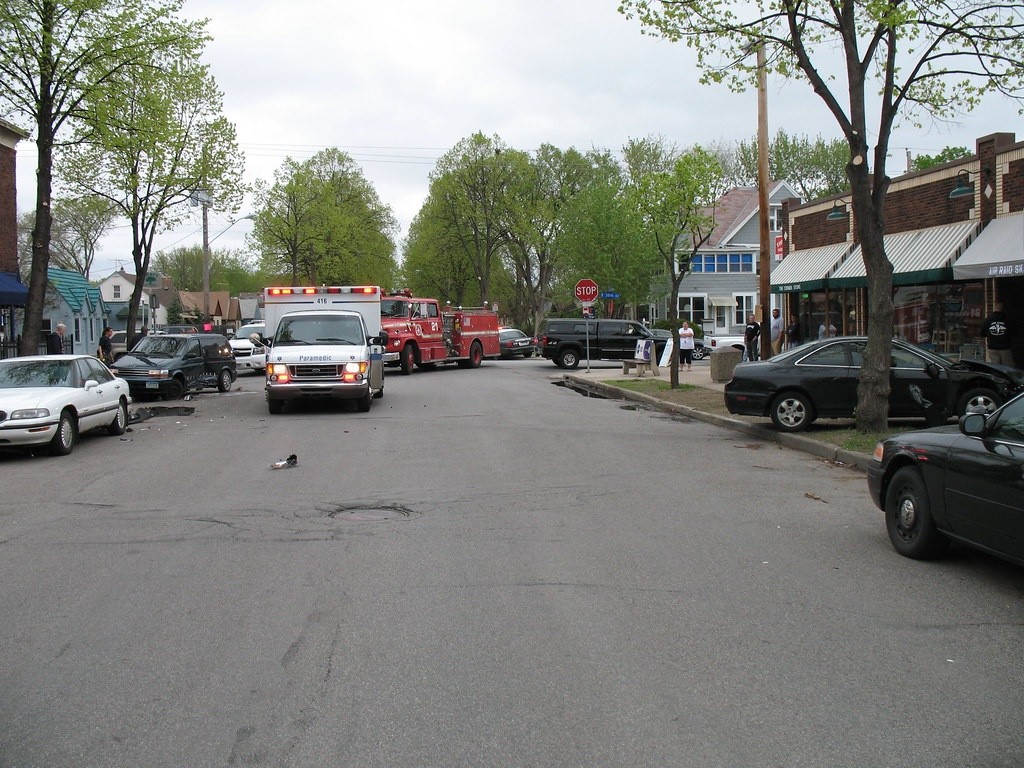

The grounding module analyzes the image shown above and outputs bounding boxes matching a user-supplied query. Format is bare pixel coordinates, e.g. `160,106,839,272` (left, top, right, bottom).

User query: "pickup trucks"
704,321,785,362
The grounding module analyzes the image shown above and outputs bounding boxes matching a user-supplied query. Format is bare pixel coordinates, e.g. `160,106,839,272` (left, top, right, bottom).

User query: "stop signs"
575,279,598,303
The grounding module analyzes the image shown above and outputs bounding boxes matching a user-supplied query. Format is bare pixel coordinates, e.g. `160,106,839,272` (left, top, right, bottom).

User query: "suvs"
158,326,200,334
108,329,168,364
534,317,673,369
108,333,237,401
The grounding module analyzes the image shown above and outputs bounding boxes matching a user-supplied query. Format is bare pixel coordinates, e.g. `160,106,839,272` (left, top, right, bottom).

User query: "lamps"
949,167,992,199
827,199,854,221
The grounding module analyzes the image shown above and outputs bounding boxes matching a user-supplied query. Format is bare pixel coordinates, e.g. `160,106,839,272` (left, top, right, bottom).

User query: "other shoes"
678,368,683,371
687,367,692,371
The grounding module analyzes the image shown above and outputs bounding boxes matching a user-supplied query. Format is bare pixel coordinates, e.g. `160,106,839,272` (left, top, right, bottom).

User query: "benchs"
622,339,661,378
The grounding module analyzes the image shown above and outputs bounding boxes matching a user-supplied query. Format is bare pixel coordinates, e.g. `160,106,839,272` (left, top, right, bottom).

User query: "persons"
130,326,150,350
981,303,1014,367
744,314,760,361
771,309,784,355
679,321,694,371
819,316,837,340
786,315,800,349
47,324,66,354
98,327,114,367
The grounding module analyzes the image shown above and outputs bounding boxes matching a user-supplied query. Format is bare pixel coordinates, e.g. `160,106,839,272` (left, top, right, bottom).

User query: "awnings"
708,297,738,306
0,273,29,308
953,214,1024,280
828,218,981,288
770,243,853,294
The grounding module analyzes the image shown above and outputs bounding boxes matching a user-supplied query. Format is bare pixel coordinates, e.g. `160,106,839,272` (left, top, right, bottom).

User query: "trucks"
263,286,385,415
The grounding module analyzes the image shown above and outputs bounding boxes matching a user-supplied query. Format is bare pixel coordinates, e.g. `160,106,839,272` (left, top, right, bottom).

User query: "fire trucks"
380,287,501,376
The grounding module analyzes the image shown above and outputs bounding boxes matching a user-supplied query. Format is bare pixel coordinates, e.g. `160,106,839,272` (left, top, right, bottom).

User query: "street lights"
191,191,213,325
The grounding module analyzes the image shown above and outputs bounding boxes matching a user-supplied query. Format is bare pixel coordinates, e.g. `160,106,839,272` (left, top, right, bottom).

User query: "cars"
227,319,267,376
0,354,133,456
482,325,533,360
648,329,707,360
722,334,1024,433
867,390,1024,570
